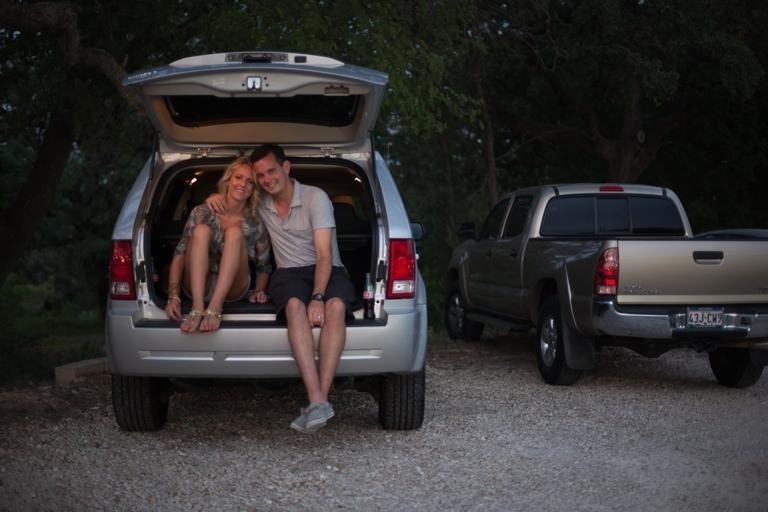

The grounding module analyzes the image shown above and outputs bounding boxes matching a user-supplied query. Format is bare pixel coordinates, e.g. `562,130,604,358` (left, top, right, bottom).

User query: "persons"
205,142,352,435
162,156,271,333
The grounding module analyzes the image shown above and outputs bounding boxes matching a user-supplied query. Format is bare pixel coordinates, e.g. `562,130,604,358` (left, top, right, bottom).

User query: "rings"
317,315,320,319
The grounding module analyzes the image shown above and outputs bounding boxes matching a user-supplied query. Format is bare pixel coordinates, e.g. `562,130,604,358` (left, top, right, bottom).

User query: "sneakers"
290,401,335,434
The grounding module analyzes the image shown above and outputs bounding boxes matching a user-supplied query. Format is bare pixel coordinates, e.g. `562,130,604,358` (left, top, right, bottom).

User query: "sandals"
180,309,222,334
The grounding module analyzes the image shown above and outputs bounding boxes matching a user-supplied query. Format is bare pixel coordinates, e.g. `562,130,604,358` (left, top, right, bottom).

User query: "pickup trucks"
438,182,768,388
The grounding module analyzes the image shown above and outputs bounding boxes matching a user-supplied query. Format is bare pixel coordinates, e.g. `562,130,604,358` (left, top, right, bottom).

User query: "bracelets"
167,283,181,304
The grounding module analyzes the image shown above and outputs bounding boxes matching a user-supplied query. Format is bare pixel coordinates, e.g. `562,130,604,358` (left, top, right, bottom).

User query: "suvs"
103,50,428,430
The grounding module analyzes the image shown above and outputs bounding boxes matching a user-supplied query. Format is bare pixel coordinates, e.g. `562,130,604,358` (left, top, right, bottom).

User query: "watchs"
311,293,326,302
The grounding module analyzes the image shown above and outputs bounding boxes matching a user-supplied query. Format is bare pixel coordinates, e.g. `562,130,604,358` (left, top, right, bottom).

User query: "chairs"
334,201,371,262
